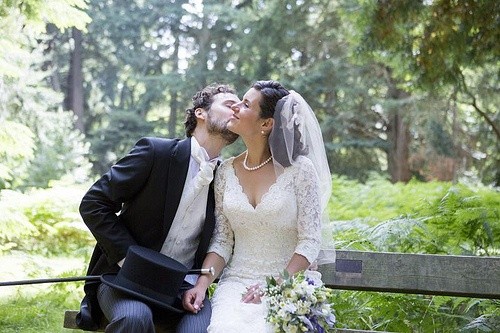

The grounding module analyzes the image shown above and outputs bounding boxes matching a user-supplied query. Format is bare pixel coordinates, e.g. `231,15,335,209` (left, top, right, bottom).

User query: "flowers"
250,268,336,333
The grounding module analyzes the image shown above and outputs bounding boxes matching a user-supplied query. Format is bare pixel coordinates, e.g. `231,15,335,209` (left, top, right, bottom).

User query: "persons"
75,80,243,333
180,80,337,333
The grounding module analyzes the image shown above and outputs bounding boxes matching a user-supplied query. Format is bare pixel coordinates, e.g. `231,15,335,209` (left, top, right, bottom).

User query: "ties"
191,136,220,200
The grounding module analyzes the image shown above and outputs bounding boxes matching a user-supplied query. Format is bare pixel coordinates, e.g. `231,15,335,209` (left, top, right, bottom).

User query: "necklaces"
245,149,273,169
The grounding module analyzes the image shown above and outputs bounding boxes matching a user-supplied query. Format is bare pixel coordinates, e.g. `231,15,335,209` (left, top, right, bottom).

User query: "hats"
99,244,191,312
